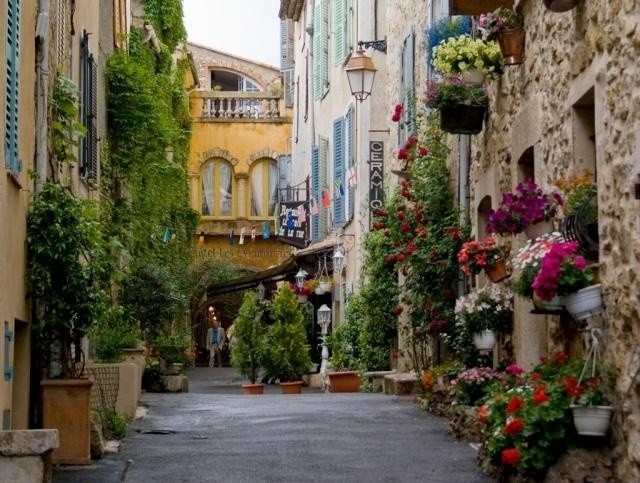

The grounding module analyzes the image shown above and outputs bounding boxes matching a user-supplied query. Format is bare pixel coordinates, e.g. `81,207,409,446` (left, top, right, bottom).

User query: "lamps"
345,38,387,103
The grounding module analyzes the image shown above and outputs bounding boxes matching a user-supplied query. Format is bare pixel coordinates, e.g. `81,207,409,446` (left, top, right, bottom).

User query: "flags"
164,166,358,253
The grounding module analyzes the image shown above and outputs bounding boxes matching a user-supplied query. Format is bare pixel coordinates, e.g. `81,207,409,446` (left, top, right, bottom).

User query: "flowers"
369,8,613,479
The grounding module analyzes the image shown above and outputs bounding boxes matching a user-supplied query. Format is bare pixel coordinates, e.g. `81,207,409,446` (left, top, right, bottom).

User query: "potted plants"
28,180,361,464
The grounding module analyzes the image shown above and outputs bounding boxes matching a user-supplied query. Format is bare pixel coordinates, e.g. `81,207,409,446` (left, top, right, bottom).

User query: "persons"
206,319,226,368
